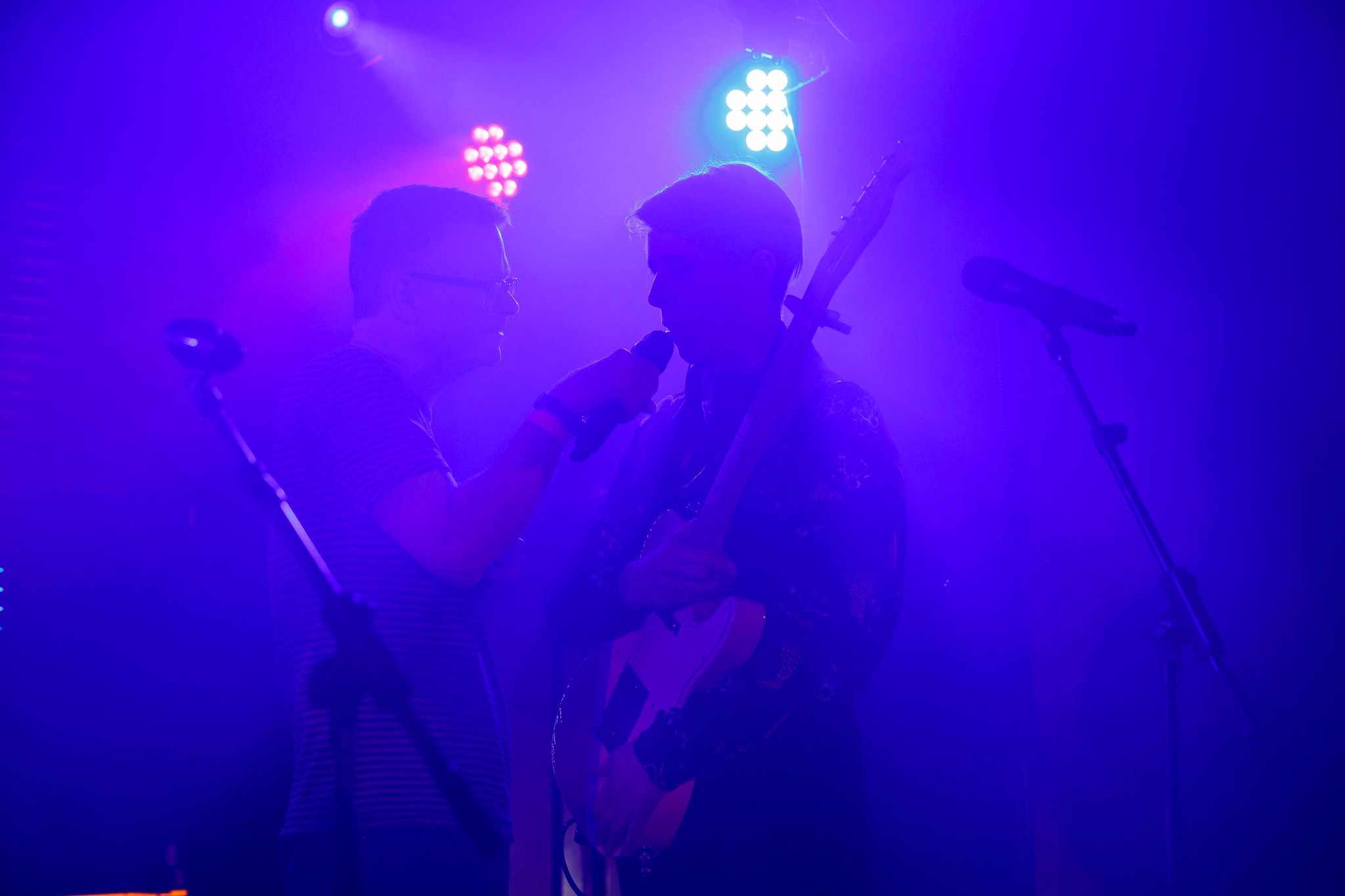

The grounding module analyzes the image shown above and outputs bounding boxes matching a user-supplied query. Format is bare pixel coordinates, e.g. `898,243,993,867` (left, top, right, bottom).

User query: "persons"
538,159,912,895
262,182,666,895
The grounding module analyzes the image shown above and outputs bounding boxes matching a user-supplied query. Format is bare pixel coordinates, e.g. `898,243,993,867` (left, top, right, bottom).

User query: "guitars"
548,115,943,875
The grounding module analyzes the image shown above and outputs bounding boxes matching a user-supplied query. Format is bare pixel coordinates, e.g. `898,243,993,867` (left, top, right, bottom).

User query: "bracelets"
527,409,573,448
534,391,586,436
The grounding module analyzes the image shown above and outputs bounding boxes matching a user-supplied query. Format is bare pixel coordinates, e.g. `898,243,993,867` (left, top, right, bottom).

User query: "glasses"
403,269,517,309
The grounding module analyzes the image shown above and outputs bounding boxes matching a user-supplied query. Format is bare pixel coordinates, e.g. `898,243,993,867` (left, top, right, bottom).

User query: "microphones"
961,255,1137,339
570,330,675,463
